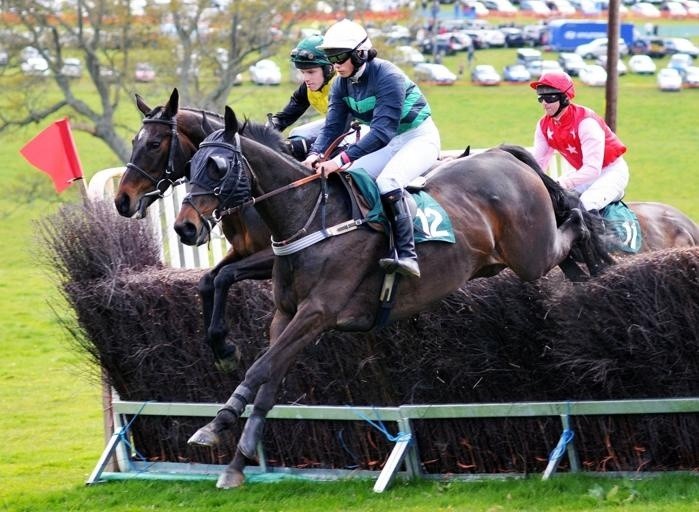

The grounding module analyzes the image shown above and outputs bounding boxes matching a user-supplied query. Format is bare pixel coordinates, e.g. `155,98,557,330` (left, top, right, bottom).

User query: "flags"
18,116,83,194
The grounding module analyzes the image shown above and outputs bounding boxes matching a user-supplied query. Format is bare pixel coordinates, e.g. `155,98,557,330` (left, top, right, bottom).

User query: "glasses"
538,94,560,102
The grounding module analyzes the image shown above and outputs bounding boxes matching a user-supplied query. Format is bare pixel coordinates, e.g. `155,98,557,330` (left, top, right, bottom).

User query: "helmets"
529,71,575,100
290,19,372,69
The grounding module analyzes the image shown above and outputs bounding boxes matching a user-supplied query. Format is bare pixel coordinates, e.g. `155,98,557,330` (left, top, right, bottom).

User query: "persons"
263,34,371,160
301,18,441,279
531,71,629,251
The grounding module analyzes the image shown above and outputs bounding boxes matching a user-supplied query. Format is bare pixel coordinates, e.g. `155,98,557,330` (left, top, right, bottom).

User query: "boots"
378,188,420,279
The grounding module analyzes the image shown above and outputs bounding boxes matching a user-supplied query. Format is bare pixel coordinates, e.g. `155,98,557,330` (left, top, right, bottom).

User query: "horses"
171,103,618,493
438,144,699,265
113,86,471,376
